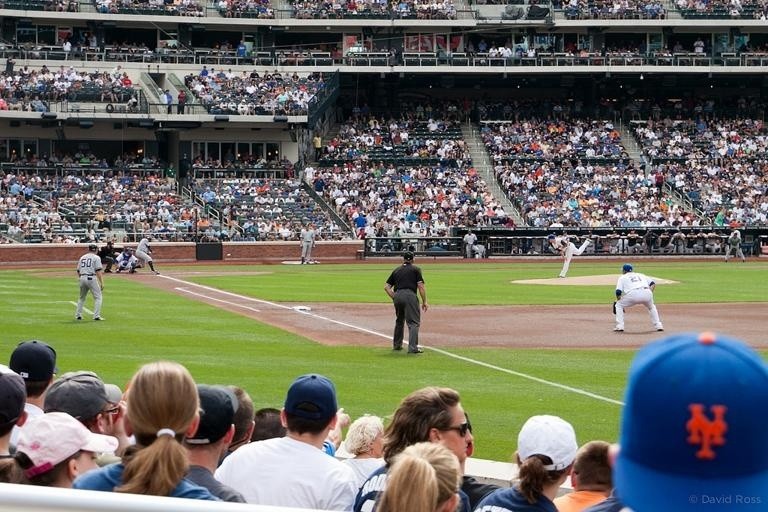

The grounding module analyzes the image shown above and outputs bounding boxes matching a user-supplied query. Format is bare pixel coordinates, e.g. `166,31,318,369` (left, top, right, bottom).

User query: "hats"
403,252,414,263
546,235,556,240
518,415,579,472
623,263,633,273
0,363,27,441
88,243,98,252
15,411,122,478
283,373,334,421
184,383,239,447
10,339,59,381
145,235,152,240
43,368,123,422
614,334,766,511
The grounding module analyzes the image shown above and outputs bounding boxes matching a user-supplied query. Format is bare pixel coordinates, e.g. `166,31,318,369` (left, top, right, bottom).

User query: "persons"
384,251,429,354
612,263,664,333
74,243,105,321
0,0,768,279
0,331,767,512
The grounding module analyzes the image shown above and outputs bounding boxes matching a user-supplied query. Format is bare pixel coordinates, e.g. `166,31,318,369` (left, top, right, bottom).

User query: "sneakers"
77,317,106,321
393,346,425,354
300,257,311,264
556,275,565,278
613,328,665,333
586,237,596,242
104,269,136,274
725,259,746,263
151,270,160,274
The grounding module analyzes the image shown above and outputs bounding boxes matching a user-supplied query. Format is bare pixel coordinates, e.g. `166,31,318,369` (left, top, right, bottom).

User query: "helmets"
125,247,133,255
107,241,116,250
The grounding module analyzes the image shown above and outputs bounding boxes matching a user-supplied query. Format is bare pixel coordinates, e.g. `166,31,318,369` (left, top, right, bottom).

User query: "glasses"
441,423,472,438
